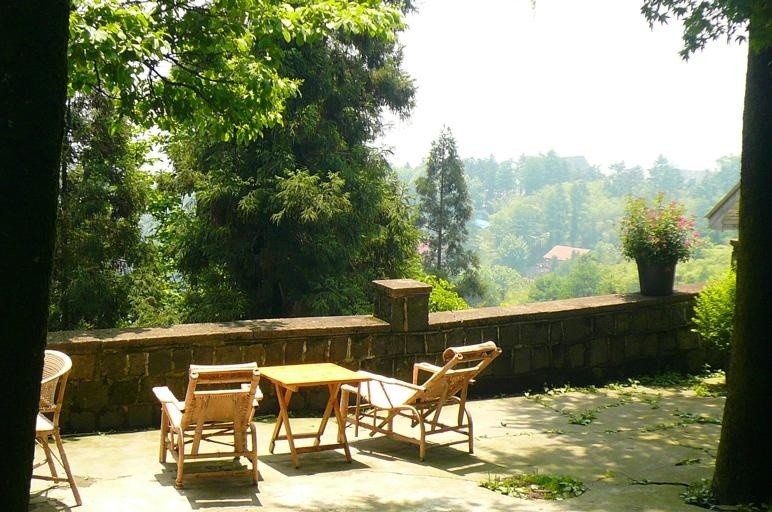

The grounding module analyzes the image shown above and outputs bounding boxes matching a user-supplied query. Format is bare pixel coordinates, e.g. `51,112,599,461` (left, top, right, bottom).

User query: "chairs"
30,350,82,506
152,362,263,490
337,341,502,462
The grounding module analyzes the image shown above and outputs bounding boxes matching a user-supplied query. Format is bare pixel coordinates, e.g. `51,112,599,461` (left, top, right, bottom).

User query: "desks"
256,363,371,470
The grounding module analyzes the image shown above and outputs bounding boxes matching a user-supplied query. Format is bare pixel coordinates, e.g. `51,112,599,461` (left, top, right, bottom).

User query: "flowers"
616,191,701,263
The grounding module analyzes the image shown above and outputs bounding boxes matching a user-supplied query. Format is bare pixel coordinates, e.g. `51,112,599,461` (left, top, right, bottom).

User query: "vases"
636,258,678,296
